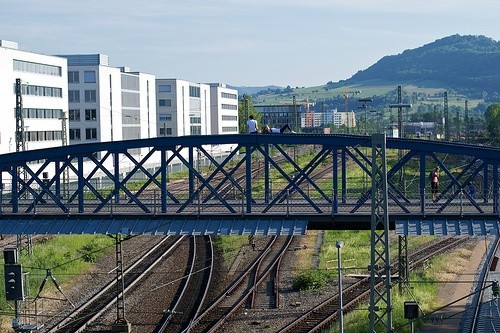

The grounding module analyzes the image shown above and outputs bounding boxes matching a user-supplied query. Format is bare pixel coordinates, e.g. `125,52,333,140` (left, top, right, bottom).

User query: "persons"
261,124,296,132
428,167,440,202
246,114,260,132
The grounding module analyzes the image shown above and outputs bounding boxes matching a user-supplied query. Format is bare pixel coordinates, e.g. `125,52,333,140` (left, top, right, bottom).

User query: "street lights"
336,240,346,333
